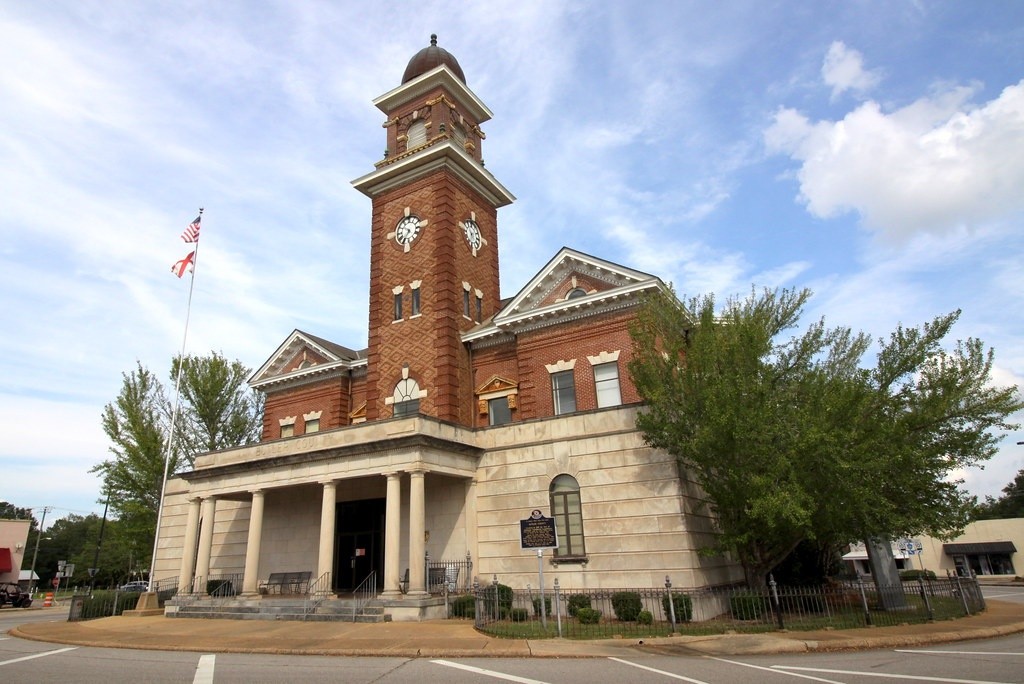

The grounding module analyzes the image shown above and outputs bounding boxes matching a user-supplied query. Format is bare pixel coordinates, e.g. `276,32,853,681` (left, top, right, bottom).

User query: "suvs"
0,582,31,608
121,581,148,591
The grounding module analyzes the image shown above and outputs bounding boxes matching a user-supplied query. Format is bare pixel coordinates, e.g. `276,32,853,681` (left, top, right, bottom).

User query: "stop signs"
53,577,59,586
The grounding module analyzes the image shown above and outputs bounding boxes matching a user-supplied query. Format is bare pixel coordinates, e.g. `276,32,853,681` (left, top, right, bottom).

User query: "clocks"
464,222,480,249
397,217,420,244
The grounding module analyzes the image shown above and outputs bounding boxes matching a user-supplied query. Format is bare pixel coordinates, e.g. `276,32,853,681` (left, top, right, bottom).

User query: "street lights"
53,564,71,607
88,480,114,595
29,537,52,594
62,560,74,606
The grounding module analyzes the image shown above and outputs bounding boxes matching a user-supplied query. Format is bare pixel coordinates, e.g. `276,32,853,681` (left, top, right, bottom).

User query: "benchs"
399,567,460,595
259,571,312,595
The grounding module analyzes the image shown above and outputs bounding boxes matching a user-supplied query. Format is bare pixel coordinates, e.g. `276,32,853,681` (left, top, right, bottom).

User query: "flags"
180,216,201,243
171,251,197,280
